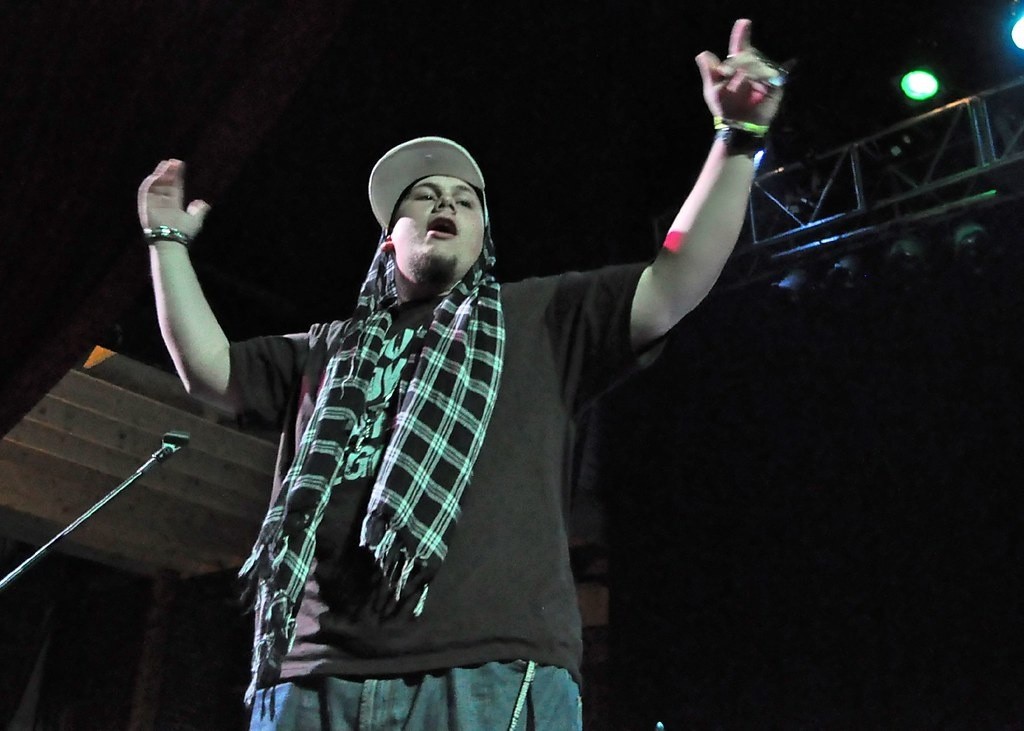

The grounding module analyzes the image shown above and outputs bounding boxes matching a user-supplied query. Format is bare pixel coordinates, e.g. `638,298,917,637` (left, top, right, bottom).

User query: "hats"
368,135,489,236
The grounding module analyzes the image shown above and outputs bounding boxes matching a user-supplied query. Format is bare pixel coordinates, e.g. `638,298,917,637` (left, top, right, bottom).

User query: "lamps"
777,268,810,306
953,219,989,256
833,252,866,280
891,235,924,272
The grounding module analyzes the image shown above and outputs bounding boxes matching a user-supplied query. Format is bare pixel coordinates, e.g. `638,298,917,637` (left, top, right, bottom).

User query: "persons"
139,18,783,731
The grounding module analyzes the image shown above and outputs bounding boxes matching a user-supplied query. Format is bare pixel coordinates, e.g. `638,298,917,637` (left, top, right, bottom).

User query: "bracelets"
712,116,770,150
143,226,191,247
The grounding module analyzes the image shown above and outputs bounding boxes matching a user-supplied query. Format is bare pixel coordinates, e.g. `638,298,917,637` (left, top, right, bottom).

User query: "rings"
751,48,788,87
